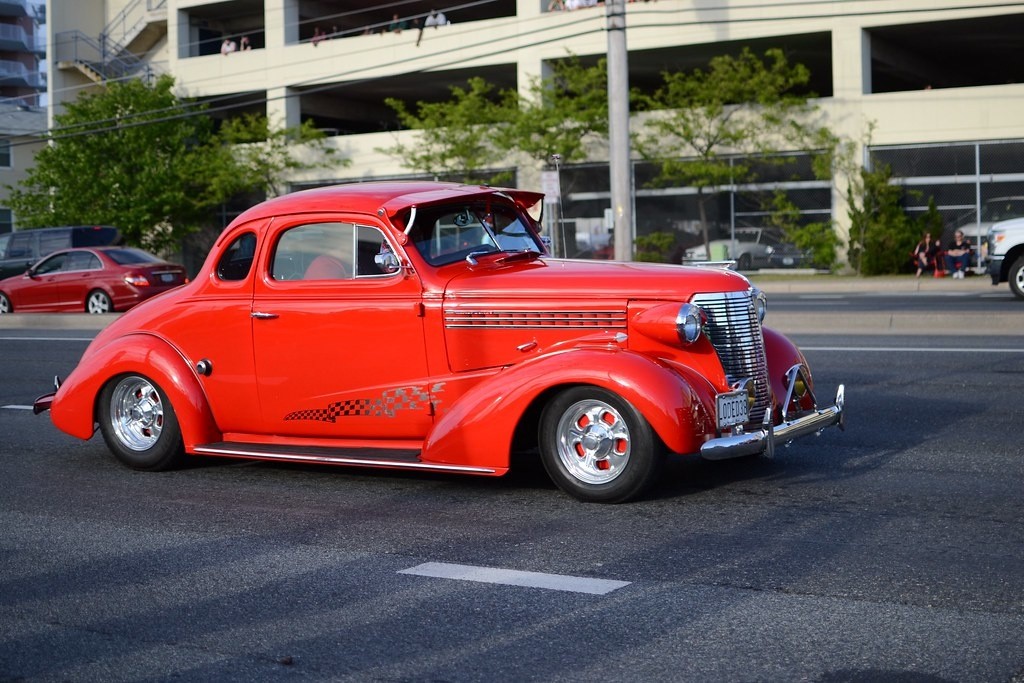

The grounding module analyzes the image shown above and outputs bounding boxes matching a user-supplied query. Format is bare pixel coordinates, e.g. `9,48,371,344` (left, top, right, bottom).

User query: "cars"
0,247,188,314
955,196,1024,265
33,182,846,505
986,217,1024,300
681,227,793,270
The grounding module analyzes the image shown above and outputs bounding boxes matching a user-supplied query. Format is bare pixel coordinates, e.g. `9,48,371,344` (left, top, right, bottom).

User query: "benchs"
909,249,974,277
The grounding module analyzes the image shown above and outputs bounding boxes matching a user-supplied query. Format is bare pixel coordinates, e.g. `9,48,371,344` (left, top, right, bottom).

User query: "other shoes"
953,272,964,279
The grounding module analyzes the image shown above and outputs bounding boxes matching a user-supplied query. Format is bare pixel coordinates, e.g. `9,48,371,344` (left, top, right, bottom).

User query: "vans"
0,225,117,280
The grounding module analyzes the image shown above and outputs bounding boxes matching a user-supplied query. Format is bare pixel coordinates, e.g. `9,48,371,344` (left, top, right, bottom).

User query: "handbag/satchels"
934,269,945,278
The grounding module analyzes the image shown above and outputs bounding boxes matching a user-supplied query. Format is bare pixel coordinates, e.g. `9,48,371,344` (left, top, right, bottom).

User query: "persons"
302,225,357,281
913,229,990,281
